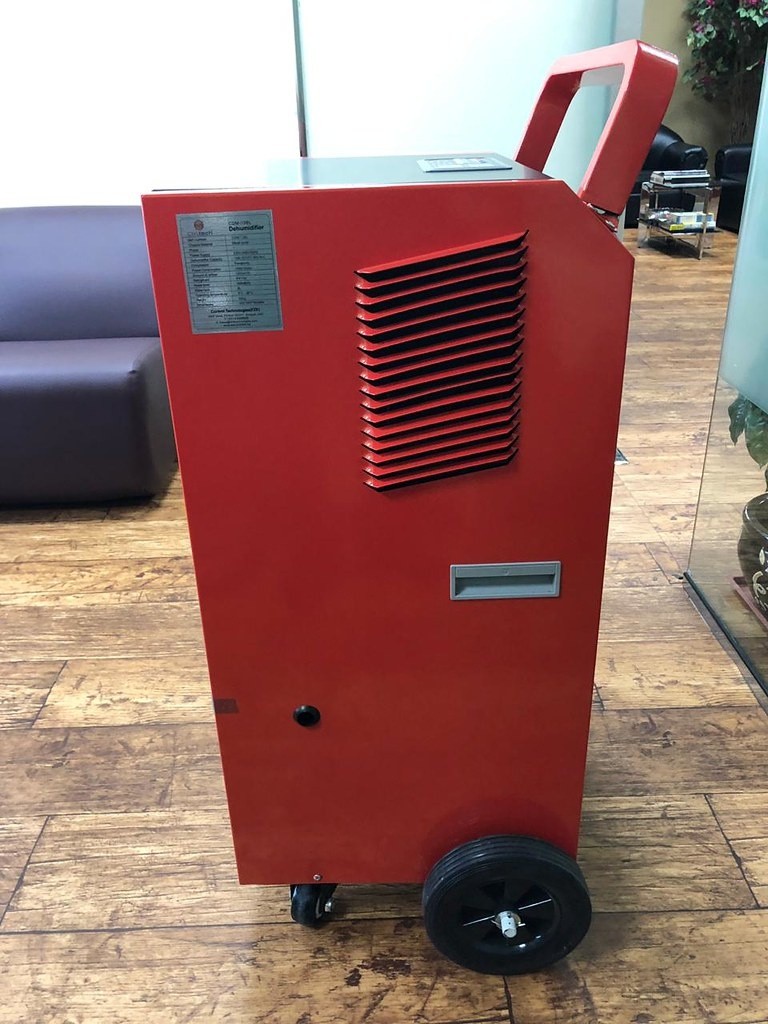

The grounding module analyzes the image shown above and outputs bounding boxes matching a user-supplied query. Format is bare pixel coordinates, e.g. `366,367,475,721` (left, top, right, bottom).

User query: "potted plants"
723,392,768,618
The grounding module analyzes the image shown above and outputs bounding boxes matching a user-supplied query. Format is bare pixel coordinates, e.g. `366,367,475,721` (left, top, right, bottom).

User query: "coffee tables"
636,181,725,260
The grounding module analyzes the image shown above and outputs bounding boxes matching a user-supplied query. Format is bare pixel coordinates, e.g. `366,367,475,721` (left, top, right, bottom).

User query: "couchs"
624,120,709,228
715,144,751,232
0,206,176,505
